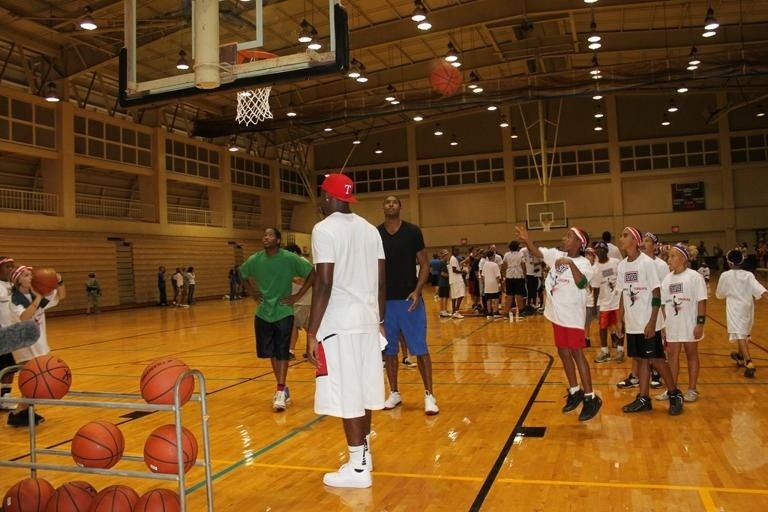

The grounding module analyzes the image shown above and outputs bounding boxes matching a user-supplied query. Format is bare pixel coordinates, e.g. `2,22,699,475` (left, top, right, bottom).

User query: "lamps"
45,81,60,102
80,5,98,31
228,137,240,152
176,27,190,70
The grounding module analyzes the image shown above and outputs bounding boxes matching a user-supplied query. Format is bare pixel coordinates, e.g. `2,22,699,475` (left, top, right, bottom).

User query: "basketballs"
18,353,72,400
32,267,59,296
143,425,198,474
139,357,194,406
2,478,181,511
429,60,462,93
71,421,124,469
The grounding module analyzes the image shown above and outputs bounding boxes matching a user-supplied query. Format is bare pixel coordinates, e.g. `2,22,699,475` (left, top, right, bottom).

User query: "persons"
373,195,442,417
282,244,315,359
156,263,197,308
229,269,237,301
4,264,66,427
84,272,102,315
422,221,766,421
234,265,242,299
380,326,418,369
236,228,317,411
0,255,22,409
305,173,388,490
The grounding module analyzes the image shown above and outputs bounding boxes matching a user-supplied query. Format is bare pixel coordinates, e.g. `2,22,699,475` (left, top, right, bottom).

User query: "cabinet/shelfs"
0,365,213,512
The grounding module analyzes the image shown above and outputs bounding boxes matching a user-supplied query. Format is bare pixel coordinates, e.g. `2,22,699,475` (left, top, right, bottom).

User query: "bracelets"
32,302,37,309
306,332,316,336
379,319,385,324
56,278,65,286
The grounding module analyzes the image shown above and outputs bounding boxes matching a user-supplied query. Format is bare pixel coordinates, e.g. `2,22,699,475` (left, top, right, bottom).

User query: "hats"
321,172,359,204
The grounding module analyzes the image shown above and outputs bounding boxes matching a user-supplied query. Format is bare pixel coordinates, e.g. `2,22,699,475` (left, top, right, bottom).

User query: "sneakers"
424,395,439,415
562,389,584,413
273,391,286,409
382,391,402,410
323,463,372,488
401,355,418,368
364,453,374,472
440,305,545,320
159,301,196,307
585,336,592,347
230,295,249,300
622,393,652,413
683,390,699,402
617,367,663,388
578,395,602,421
730,350,756,377
272,387,290,401
667,390,684,415
610,333,620,348
1,394,45,426
596,345,623,364
654,389,669,400
